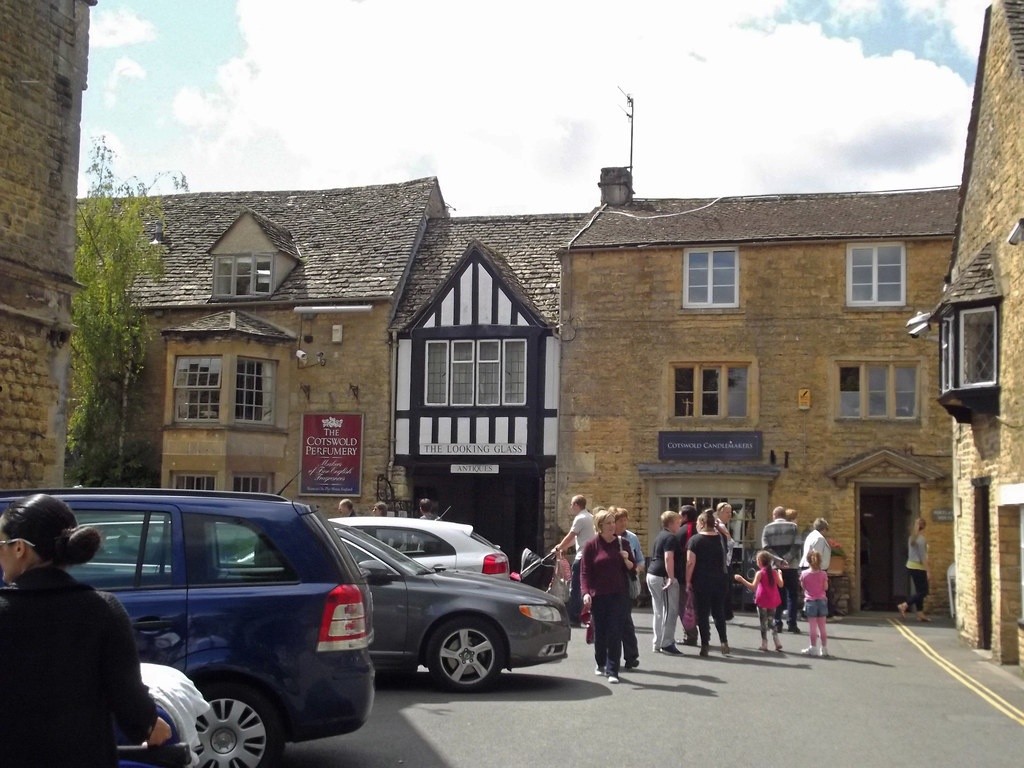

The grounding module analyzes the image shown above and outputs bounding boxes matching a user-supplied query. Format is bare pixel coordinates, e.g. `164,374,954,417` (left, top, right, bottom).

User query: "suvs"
0,485,378,768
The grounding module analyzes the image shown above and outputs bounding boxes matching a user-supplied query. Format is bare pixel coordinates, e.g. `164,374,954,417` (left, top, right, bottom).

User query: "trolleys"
110,696,200,768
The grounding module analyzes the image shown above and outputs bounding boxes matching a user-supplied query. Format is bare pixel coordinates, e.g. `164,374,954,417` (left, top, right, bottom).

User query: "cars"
57,508,573,695
232,515,512,584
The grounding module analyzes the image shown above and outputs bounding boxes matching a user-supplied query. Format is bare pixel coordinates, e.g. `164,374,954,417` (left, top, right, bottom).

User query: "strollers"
509,547,564,584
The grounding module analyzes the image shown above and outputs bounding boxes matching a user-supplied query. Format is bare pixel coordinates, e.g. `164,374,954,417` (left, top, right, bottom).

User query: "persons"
799,516,841,622
646,502,735,656
897,519,933,622
338,498,356,517
0,493,173,768
419,498,442,520
550,495,646,683
373,502,387,516
798,550,830,656
733,550,785,651
763,505,801,633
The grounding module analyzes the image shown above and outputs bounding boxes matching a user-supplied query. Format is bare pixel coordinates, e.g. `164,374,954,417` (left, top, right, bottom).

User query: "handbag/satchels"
618,536,641,601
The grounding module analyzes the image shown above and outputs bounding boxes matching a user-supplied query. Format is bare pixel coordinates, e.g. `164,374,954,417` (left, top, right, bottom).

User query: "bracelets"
556,548,558,551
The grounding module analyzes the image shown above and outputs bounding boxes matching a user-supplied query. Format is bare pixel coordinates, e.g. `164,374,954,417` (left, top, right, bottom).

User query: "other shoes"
625,660,639,669
819,647,829,656
759,647,768,651
721,640,730,656
917,617,932,623
775,645,782,650
788,626,800,633
653,649,661,653
898,604,906,620
609,676,619,684
700,644,710,657
677,639,697,646
660,645,683,656
801,646,819,656
595,665,604,676
775,626,782,633
